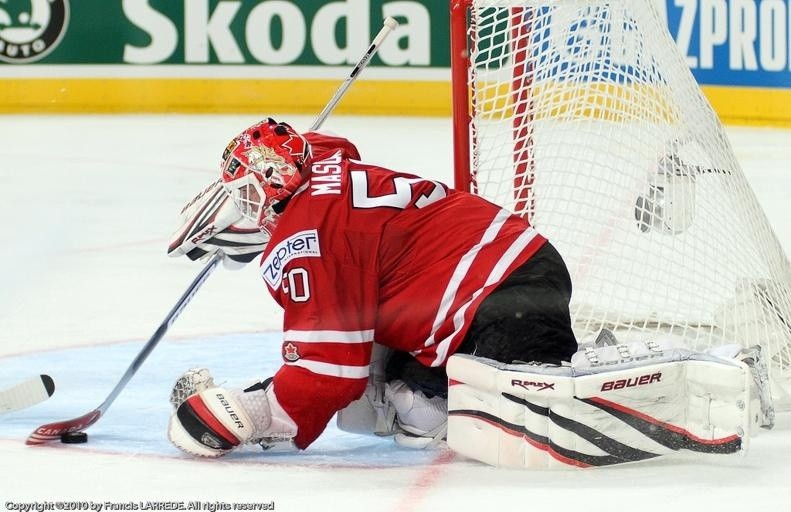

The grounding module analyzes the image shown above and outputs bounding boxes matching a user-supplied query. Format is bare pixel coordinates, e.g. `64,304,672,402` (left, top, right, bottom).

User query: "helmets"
222,118,315,237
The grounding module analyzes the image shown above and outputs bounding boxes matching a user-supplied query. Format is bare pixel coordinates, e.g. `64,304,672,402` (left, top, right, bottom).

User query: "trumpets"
22,17,399,447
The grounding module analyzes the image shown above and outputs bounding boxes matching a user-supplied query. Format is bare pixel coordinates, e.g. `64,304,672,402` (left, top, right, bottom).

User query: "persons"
161,114,753,460
